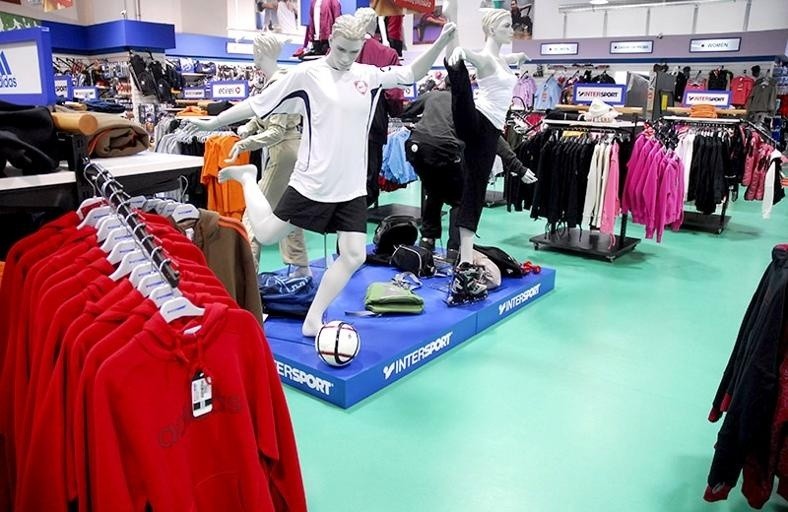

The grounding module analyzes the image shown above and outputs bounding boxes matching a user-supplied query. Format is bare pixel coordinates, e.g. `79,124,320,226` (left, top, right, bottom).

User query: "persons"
226,30,315,291
444,8,530,272
405,76,538,264
509,0,530,31
180,14,456,339
352,6,404,210
253,0,449,62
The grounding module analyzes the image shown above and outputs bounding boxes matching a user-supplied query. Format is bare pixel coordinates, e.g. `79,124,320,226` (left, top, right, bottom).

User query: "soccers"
314,320,361,367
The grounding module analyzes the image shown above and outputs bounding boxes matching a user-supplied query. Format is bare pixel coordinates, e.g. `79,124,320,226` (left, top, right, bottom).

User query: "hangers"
389,116,411,135
671,66,773,86
156,115,241,142
70,159,207,329
507,111,782,153
519,66,609,79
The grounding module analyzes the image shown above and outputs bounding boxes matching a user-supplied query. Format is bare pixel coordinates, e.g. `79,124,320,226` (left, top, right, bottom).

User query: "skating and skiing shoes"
446,261,488,307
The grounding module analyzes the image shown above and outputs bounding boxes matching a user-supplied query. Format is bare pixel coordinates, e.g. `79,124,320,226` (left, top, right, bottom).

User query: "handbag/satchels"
473,250,501,289
373,215,418,256
194,62,217,74
473,243,523,278
364,282,424,315
388,242,435,278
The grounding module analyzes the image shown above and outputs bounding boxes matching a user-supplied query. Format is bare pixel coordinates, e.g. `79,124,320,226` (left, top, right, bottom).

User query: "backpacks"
256,273,327,320
